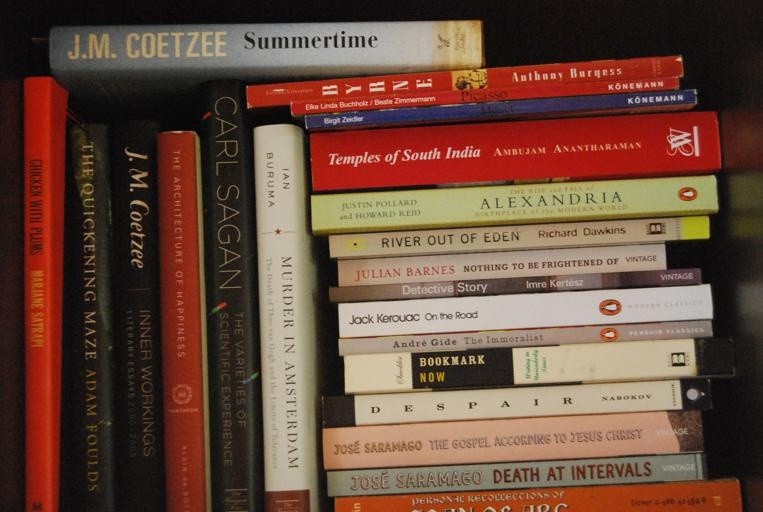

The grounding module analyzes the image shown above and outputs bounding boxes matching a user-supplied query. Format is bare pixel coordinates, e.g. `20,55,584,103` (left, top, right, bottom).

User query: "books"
16,20,761,512
